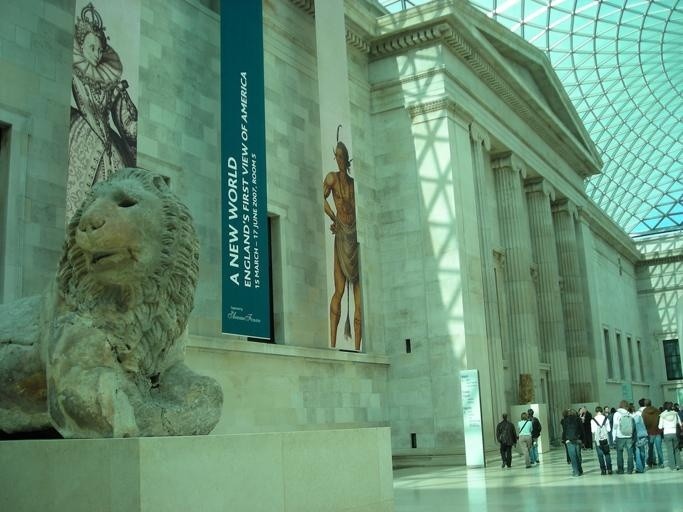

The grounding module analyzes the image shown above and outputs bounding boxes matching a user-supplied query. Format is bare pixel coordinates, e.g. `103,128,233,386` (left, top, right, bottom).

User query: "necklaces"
337,171,353,214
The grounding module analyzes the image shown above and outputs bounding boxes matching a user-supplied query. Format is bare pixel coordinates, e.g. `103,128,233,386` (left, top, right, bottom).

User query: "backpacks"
593,417,610,446
617,411,633,435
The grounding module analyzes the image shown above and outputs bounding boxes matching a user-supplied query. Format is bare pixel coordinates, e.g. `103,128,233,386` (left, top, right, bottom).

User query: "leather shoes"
601,463,664,475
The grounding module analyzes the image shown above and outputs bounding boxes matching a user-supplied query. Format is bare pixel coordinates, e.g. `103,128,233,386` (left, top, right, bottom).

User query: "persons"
323,142,362,350
561,398,683,477
527,408,541,464
518,412,533,468
496,413,517,468
64,3,138,225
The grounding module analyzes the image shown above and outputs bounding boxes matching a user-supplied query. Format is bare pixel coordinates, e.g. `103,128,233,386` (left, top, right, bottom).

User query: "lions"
0,166,223,440
